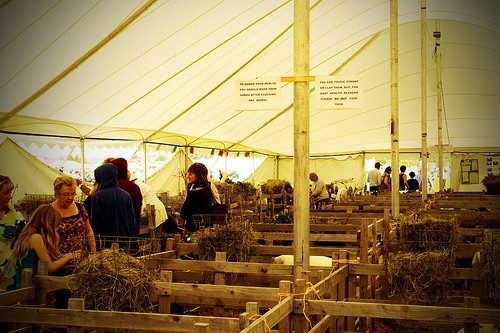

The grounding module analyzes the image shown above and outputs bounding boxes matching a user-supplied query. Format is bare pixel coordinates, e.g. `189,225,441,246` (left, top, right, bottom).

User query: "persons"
381,167,391,192
284,184,294,204
83,165,139,259
180,162,213,233
407,172,419,192
49,174,97,308
310,173,330,208
73,157,177,241
0,175,26,293
7,205,82,289
399,165,409,191
367,162,381,196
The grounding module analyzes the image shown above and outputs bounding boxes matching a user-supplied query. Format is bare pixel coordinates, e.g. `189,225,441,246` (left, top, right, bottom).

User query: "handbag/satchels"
207,188,229,225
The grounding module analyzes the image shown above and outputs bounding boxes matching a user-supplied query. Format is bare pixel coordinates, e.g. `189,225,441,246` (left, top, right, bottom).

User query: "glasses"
58,192,77,198
